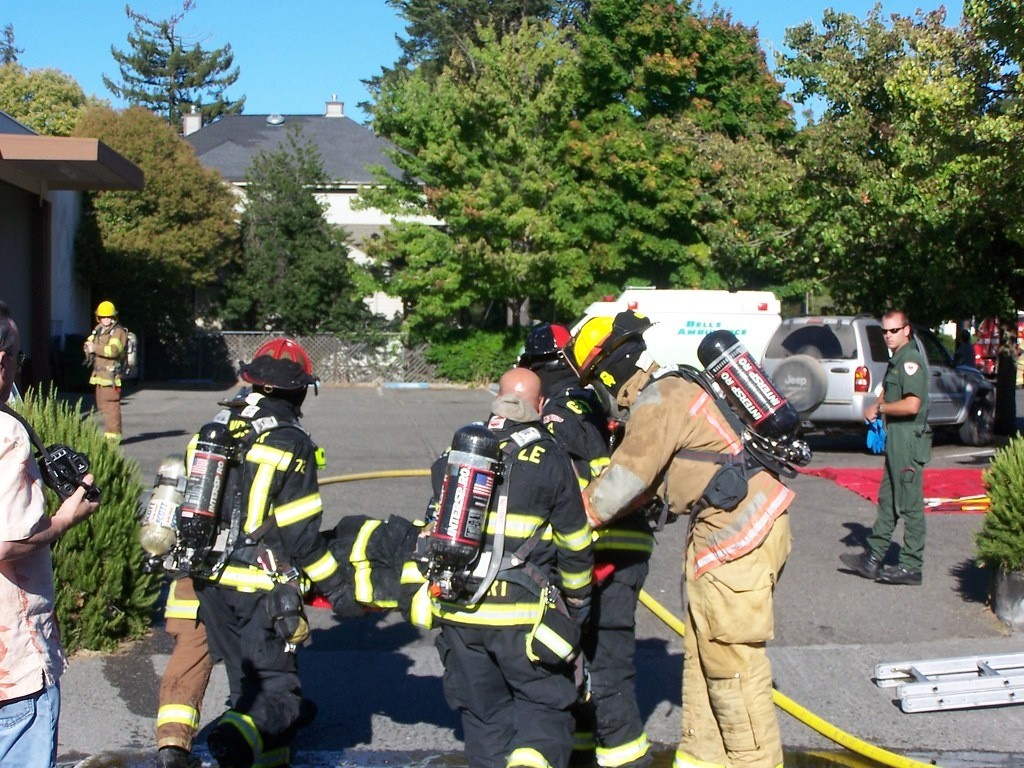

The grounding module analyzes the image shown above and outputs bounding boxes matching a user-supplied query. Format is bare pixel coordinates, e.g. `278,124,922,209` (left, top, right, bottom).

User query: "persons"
423,368,592,768
573,311,796,768
303,512,444,629
956,329,976,368
510,322,667,768
83,300,126,446
184,338,365,767
152,573,217,767
0,304,104,768
839,309,932,586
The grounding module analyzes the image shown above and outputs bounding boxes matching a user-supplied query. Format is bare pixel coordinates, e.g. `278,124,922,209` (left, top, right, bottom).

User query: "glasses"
6,350,26,365
880,325,907,334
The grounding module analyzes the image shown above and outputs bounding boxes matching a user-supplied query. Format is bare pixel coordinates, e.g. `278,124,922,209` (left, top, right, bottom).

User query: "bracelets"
877,404,882,415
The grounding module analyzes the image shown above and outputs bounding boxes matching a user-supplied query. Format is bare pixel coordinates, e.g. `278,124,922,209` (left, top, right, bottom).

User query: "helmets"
240,337,320,392
524,323,574,357
573,312,660,388
95,300,116,318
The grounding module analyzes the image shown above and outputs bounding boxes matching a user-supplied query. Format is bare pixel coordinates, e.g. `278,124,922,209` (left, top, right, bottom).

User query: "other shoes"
207,722,253,768
157,748,187,768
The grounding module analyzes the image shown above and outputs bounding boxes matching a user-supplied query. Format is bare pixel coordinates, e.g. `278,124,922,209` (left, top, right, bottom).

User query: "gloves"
865,418,887,454
325,585,369,619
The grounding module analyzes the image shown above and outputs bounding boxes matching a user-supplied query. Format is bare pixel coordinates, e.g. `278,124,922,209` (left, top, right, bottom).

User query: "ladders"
874,649,1024,714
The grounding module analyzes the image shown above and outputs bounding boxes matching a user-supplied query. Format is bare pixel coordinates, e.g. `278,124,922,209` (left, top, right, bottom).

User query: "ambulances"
569,285,781,382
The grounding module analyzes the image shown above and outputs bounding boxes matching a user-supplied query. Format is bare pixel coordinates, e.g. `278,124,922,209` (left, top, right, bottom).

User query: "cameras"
37,445,102,504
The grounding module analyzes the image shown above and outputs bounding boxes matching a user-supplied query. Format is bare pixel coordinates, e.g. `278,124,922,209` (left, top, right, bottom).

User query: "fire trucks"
972,311,1024,373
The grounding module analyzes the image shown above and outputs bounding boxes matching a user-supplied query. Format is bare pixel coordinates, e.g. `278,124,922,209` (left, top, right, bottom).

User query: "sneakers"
878,562,921,585
839,551,881,580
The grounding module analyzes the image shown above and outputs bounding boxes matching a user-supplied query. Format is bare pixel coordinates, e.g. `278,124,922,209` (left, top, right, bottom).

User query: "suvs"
762,316,997,448
